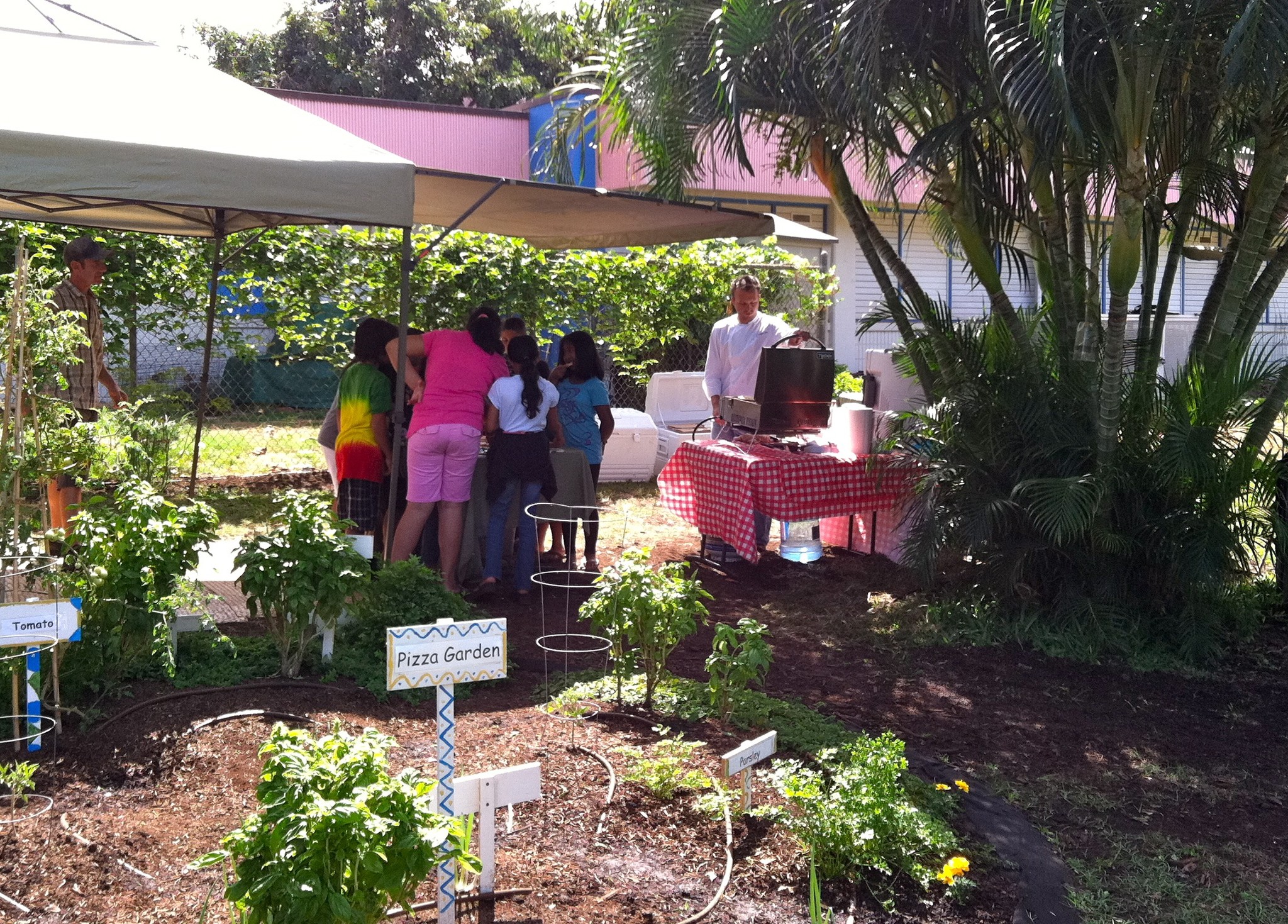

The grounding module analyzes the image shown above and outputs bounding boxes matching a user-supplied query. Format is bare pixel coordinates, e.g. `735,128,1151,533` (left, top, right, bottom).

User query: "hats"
64,237,112,267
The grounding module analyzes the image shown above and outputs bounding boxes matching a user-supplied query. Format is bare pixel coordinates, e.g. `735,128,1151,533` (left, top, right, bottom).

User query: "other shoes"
457,585,479,601
566,553,576,564
520,594,534,605
482,583,498,595
705,535,724,545
585,557,599,570
757,545,766,553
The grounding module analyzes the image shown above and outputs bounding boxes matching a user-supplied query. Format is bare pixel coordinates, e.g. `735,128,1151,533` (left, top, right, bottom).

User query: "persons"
20,238,128,557
702,273,811,560
318,304,614,606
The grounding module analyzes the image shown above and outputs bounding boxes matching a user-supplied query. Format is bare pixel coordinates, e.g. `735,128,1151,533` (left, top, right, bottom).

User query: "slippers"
542,550,567,563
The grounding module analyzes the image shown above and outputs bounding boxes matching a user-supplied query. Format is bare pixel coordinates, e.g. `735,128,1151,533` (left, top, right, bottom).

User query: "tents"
762,210,840,250
0,0,775,581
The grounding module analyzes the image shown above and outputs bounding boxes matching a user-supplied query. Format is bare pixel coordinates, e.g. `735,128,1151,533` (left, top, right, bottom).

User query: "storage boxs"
644,370,713,477
595,407,658,483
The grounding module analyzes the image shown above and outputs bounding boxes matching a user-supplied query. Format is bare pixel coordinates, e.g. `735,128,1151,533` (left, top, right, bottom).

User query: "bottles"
780,519,822,564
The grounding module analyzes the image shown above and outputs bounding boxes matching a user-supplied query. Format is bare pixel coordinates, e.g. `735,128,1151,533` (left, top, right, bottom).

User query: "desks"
417,443,596,584
657,439,931,585
